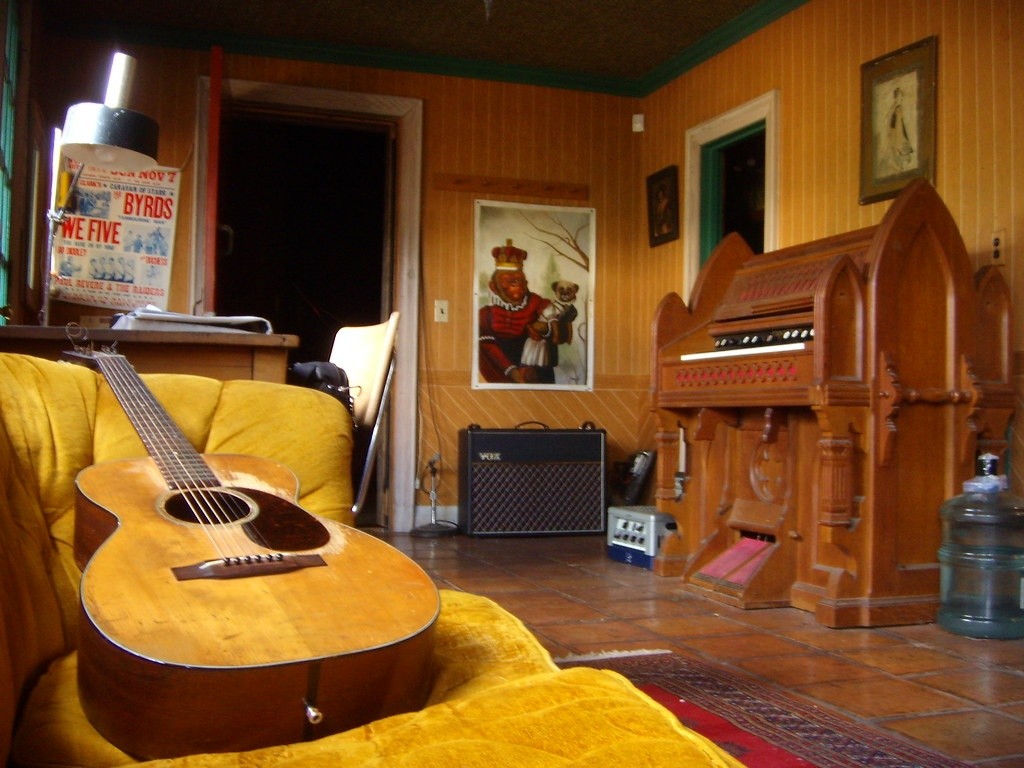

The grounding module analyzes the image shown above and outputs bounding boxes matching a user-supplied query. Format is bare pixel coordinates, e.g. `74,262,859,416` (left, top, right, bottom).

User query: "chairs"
329,312,401,507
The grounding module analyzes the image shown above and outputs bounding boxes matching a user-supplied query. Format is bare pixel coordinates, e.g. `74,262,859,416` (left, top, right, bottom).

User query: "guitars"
63,338,443,762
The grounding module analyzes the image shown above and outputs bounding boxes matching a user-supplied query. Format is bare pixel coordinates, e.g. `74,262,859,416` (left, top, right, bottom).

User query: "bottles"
936,454,1024,640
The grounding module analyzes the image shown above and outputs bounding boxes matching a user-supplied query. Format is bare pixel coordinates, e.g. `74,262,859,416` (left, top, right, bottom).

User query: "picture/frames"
646,165,679,246
472,199,596,392
857,35,937,205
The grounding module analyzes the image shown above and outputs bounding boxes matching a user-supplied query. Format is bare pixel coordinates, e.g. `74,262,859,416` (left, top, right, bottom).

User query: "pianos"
649,177,1010,629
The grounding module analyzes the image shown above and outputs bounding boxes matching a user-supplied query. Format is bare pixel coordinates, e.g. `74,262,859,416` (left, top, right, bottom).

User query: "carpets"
552,649,973,768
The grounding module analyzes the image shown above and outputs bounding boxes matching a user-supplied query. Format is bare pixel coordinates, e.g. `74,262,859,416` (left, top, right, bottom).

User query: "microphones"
427,454,441,468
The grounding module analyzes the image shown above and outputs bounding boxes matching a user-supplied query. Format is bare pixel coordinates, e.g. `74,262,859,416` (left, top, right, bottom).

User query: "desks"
0,326,300,385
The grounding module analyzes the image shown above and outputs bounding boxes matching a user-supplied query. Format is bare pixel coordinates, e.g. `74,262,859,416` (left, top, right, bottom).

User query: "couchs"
0,354,741,768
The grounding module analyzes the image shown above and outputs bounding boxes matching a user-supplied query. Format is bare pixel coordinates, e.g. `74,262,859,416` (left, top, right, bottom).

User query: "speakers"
456,428,607,537
